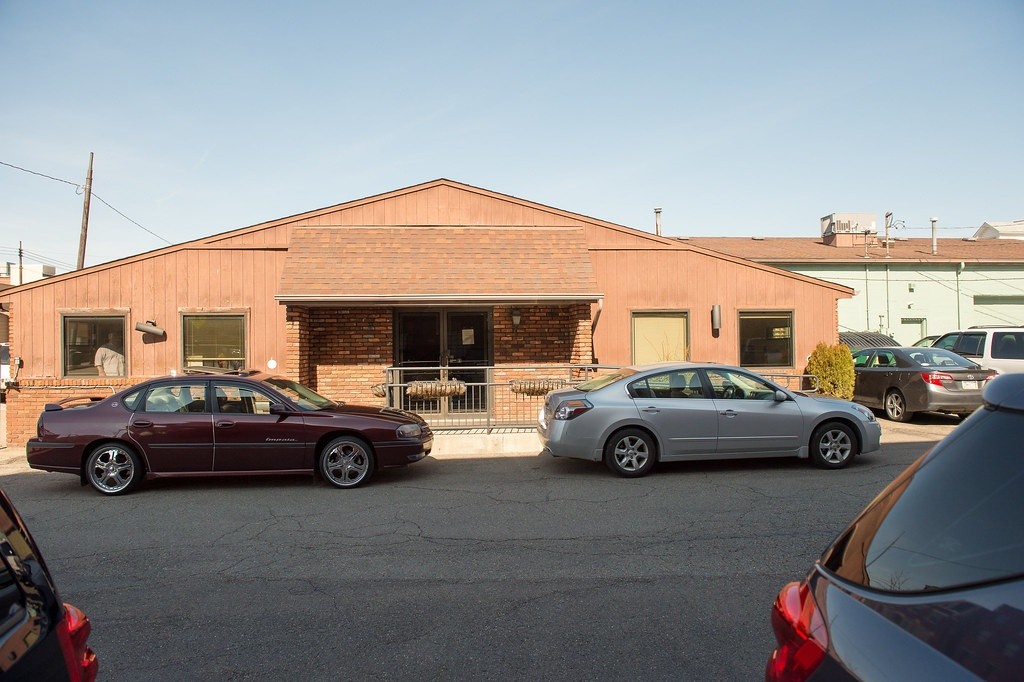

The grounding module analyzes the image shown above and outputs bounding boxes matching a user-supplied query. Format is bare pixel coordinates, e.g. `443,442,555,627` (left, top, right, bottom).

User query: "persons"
94,330,123,376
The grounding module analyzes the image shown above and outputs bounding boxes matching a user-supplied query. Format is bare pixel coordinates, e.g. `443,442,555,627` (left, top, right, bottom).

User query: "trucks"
0,342,10,403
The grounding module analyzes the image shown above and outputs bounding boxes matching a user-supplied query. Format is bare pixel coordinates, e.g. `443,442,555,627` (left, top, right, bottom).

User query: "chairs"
671,374,703,398
216,388,240,413
874,355,893,367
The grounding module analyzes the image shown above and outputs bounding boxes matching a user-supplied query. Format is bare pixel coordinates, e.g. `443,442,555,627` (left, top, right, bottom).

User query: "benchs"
146,396,189,412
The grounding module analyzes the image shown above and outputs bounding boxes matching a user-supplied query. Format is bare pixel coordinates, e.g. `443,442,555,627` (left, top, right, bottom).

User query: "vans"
913,328,1024,374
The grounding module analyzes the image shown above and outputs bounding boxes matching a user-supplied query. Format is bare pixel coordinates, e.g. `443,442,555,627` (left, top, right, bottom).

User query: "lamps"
712,304,721,329
135,320,164,337
510,307,523,332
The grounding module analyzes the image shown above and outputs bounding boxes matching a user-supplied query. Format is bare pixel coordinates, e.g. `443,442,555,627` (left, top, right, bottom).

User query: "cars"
764,375,1024,682
536,362,882,478
0,488,99,682
26,366,433,496
851,346,1000,423
